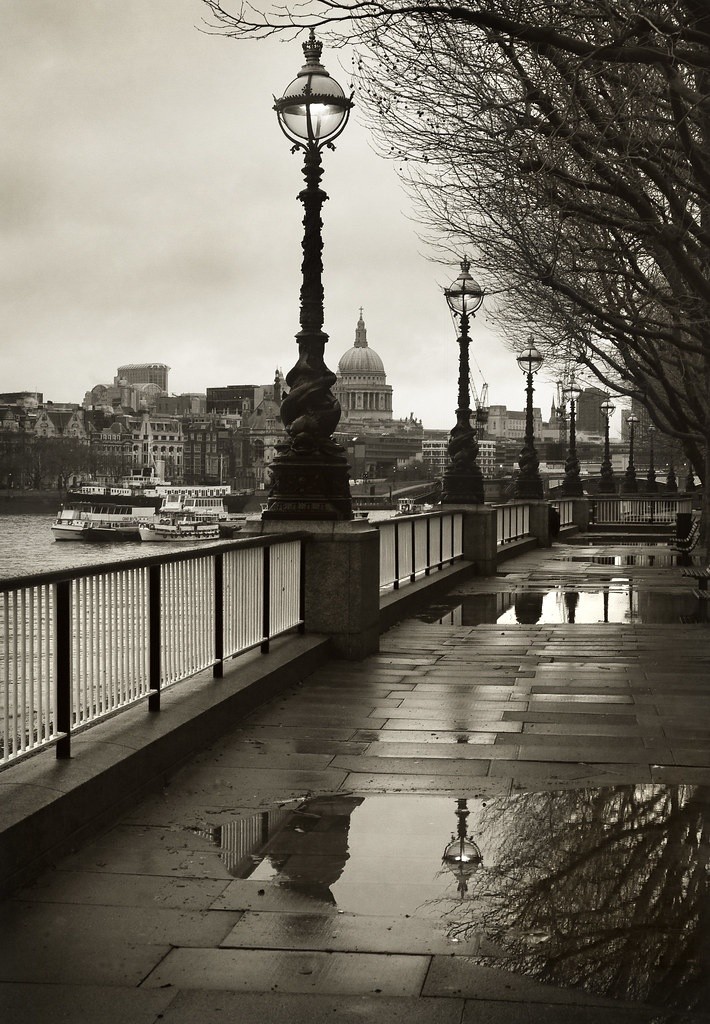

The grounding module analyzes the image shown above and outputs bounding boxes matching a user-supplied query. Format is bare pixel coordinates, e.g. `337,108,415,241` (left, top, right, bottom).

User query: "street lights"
620,412,639,493
645,423,659,492
440,254,486,506
255,25,356,522
511,331,546,499
560,371,584,497
599,394,616,493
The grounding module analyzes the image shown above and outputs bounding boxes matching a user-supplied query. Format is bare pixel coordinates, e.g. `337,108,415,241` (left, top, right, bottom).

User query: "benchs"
668,530,702,566
670,521,698,547
677,610,708,625
679,565,710,590
691,589,710,610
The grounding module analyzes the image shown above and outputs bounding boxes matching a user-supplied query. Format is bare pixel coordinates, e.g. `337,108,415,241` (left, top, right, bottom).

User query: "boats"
50,469,231,544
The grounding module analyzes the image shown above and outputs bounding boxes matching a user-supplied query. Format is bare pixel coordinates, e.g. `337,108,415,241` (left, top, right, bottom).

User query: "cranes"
443,284,490,440
